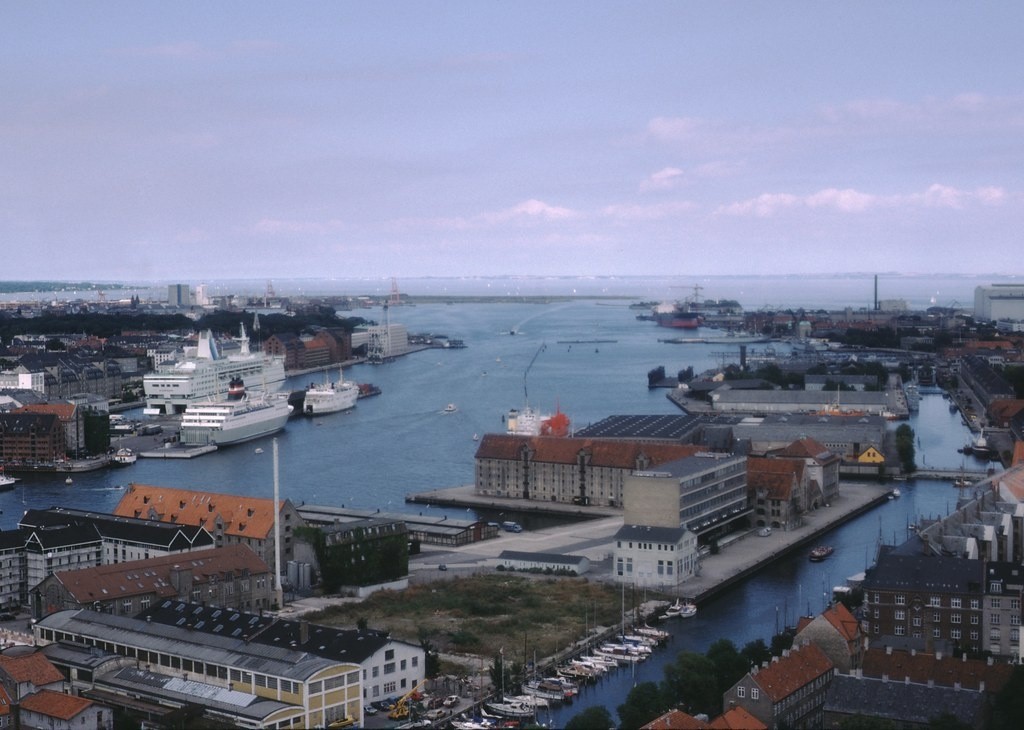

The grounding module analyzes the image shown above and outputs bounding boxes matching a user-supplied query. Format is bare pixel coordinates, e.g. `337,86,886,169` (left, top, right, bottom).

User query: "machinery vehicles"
388,678,429,721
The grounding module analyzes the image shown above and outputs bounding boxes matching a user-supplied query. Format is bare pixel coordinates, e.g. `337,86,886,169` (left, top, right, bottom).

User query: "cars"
362,694,461,729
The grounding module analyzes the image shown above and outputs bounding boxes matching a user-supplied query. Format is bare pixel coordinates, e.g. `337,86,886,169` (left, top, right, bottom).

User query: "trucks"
503,521,523,533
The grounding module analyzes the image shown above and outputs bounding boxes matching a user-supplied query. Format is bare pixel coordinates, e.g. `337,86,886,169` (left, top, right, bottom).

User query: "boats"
448,597,698,730
142,320,287,417
328,714,355,729
302,363,361,416
109,448,137,466
807,545,834,562
970,428,990,459
179,374,294,447
